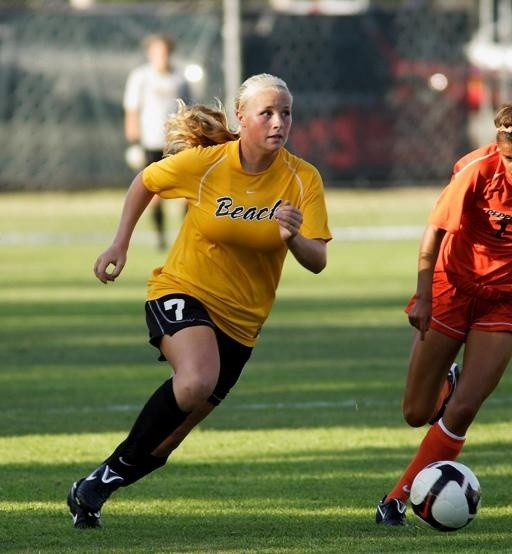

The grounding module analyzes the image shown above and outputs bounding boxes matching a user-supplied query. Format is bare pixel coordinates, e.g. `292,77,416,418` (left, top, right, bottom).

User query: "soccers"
411,460,482,532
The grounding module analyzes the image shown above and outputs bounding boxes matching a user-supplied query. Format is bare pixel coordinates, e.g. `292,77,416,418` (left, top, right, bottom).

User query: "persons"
65,70,335,533
121,30,200,255
371,101,512,525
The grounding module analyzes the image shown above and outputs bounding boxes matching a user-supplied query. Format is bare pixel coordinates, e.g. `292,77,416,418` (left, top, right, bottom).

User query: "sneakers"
374,493,408,527
67,475,103,529
75,462,124,514
428,361,462,425
